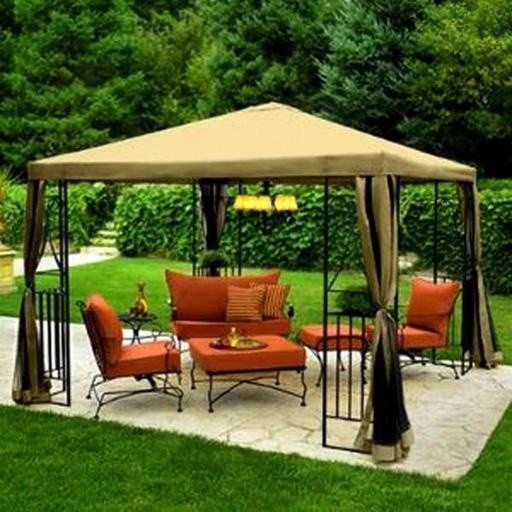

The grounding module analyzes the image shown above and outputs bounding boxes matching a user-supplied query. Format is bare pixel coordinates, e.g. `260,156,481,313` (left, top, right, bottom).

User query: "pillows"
221,281,267,323
249,279,292,320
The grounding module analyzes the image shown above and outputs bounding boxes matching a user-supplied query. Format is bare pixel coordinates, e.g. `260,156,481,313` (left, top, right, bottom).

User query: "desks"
118,311,157,345
186,333,310,413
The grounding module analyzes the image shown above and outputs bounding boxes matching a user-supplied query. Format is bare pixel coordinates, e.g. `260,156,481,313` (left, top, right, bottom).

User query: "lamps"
232,179,298,235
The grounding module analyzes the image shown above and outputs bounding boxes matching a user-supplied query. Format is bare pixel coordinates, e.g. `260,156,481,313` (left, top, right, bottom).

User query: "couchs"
73,292,183,420
296,273,464,388
161,266,296,377
